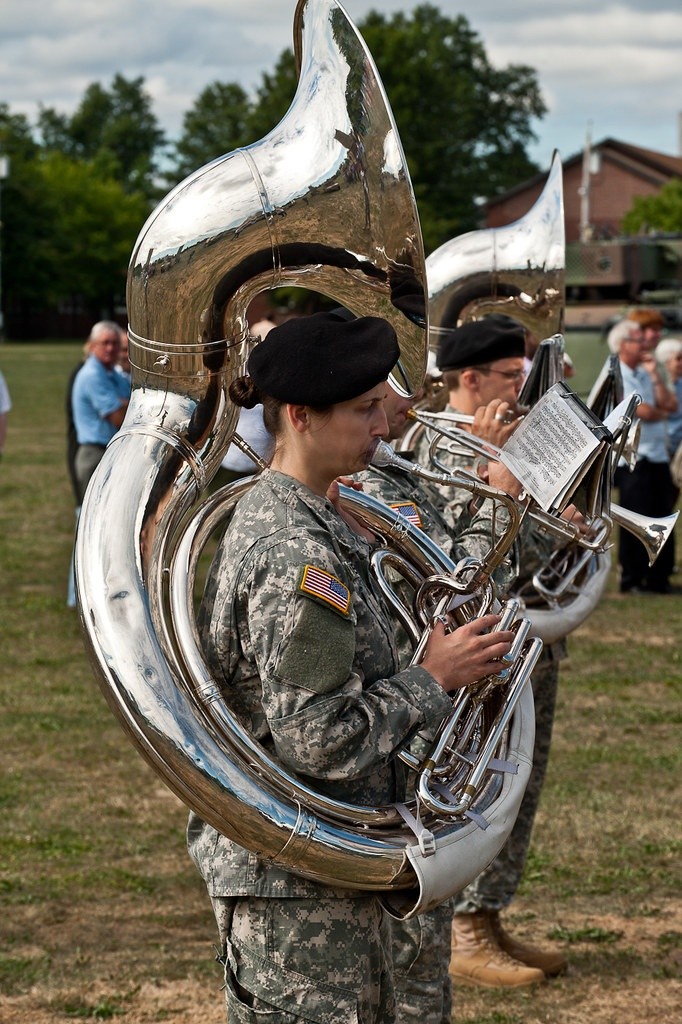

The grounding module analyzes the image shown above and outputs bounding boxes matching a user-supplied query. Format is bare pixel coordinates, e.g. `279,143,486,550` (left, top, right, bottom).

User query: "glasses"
460,367,528,383
624,337,645,343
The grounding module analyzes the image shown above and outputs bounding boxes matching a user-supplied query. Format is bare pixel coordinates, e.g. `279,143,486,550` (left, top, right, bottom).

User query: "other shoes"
638,581,682,594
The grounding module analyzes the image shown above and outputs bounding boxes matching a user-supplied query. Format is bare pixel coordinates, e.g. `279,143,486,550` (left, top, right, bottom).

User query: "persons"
0,367,12,455
607,308,682,595
64,305,587,1024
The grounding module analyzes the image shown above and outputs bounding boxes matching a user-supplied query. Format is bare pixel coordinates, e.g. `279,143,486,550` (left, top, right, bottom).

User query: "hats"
247,310,400,405
435,318,526,371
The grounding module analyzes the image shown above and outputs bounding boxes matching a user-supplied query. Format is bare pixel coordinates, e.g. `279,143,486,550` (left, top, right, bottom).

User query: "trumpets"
405,407,680,568
411,409,641,473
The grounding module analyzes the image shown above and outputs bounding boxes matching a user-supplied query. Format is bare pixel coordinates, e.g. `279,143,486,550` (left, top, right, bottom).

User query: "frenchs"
397,149,612,645
73,0,544,921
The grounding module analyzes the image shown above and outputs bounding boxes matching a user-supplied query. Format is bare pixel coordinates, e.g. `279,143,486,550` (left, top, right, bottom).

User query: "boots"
448,910,546,989
490,910,568,975
